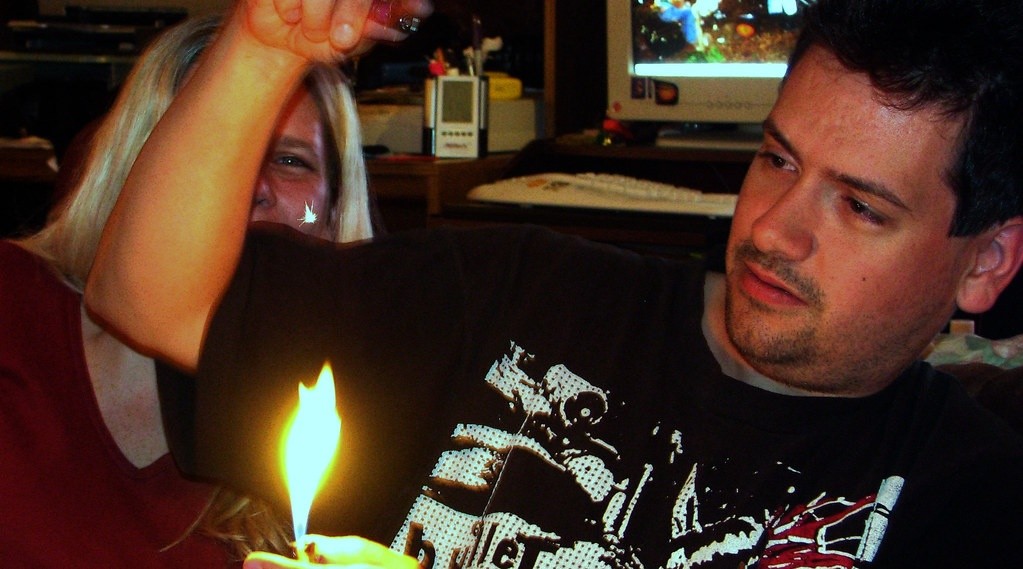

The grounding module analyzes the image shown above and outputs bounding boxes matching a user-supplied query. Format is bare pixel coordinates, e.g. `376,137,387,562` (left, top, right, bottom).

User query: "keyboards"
465,172,738,215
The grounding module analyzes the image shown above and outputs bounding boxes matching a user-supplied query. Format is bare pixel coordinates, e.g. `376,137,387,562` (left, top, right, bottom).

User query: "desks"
361,151,516,231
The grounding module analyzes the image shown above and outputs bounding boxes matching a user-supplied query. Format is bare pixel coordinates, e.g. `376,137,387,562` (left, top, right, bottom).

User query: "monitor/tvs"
607,0,817,123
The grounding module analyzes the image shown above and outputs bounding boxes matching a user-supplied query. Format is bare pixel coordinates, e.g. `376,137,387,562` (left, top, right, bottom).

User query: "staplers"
599,118,635,148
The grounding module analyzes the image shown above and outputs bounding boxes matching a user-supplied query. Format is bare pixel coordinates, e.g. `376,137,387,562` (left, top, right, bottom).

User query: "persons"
0,11,377,569
81,0,1023,569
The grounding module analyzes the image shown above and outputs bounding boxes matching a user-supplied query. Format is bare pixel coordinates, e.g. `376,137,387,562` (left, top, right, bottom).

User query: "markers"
477,77,487,157
421,77,437,157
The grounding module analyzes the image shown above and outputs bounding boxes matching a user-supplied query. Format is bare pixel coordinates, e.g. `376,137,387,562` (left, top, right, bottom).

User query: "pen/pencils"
423,12,505,77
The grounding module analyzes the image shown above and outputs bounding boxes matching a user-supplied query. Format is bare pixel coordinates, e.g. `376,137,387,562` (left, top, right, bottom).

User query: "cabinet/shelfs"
0,50,139,242
430,137,757,247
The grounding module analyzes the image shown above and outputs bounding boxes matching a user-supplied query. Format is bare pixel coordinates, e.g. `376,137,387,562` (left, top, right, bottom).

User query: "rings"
399,13,421,33
